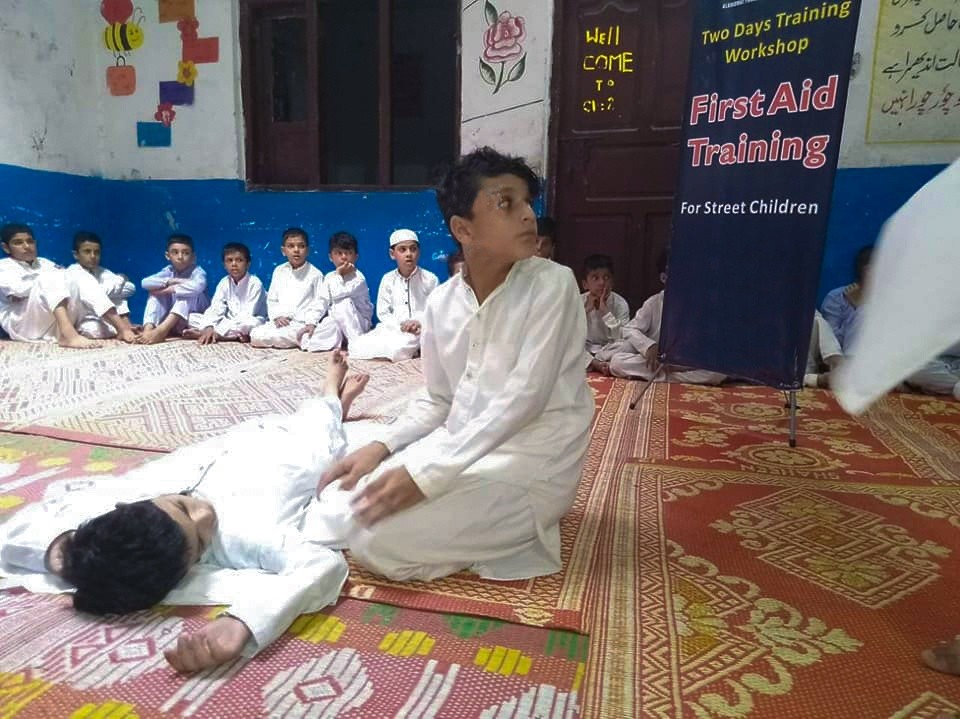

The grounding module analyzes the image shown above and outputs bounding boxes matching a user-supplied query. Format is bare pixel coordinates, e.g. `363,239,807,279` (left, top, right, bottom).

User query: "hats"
389,229,420,246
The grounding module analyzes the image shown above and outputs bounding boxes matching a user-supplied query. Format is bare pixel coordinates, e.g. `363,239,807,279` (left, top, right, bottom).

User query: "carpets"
0,328,960,719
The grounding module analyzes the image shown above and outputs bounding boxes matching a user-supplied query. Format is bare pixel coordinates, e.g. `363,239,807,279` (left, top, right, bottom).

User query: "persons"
580,253,631,377
296,230,375,354
346,228,441,364
608,246,729,386
63,230,143,344
134,233,212,345
180,241,270,346
802,244,960,402
0,222,103,349
298,146,596,583
447,250,465,277
534,216,557,262
248,226,326,350
0,348,370,673
